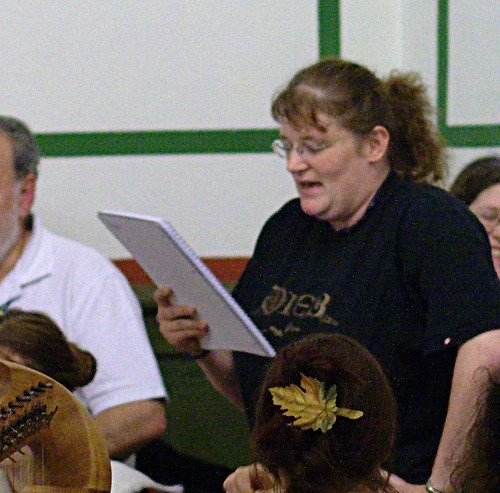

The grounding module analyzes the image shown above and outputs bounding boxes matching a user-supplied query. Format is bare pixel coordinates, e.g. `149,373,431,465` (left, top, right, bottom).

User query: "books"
99,208,276,357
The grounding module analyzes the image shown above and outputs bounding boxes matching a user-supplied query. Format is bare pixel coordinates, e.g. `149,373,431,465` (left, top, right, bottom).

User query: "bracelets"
424,478,441,493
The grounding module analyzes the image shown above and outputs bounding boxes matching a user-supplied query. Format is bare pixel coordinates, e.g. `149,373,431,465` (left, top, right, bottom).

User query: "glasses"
272,127,369,160
470,205,500,231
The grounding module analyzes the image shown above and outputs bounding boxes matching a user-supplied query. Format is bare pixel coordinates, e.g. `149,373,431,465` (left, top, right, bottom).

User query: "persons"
0,114,171,463
223,331,398,493
2,309,99,399
449,155,500,276
155,57,500,493
